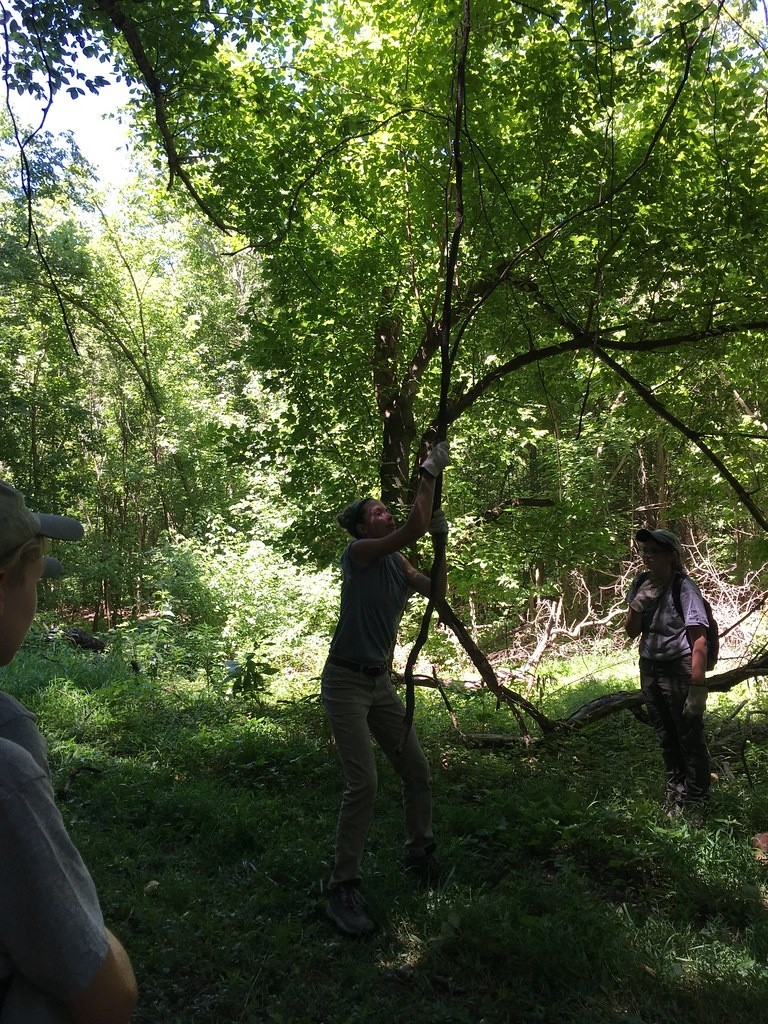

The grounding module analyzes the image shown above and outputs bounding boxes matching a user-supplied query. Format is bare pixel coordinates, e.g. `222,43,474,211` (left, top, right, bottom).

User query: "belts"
328,656,387,679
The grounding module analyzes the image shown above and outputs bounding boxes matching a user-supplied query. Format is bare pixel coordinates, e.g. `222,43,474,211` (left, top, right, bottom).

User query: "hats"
0,481,84,556
635,528,681,556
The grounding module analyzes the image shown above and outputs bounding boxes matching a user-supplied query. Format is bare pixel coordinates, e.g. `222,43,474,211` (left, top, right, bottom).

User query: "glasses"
643,546,665,554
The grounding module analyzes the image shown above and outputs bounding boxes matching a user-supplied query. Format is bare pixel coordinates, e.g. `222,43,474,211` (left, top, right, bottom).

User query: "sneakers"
326,878,374,933
409,842,444,886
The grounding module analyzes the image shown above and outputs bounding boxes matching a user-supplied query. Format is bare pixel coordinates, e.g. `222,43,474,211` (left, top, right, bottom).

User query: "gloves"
629,577,666,614
427,509,447,534
682,683,708,718
418,442,451,479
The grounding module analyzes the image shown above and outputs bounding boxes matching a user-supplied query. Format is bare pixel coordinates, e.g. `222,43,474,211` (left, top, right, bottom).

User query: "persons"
622,530,712,815
320,441,451,941
1,477,139,1024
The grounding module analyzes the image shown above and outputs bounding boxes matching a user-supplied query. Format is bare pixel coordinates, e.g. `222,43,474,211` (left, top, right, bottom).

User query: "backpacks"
636,571,718,671
